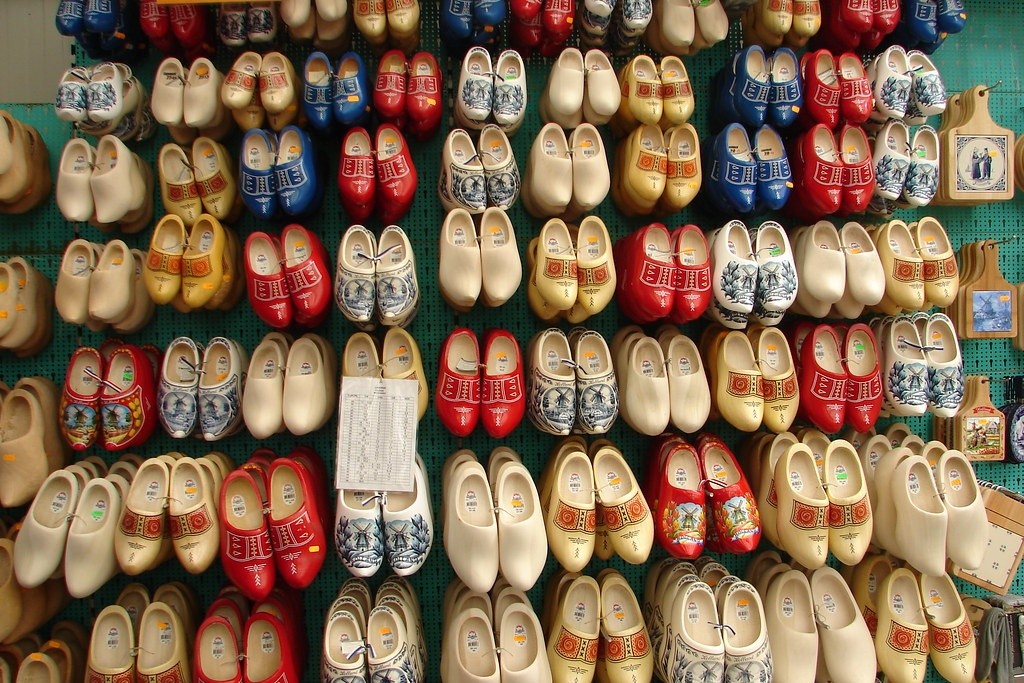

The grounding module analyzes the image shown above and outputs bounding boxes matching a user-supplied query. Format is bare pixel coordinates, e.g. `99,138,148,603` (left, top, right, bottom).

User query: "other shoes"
0,1,989,683
